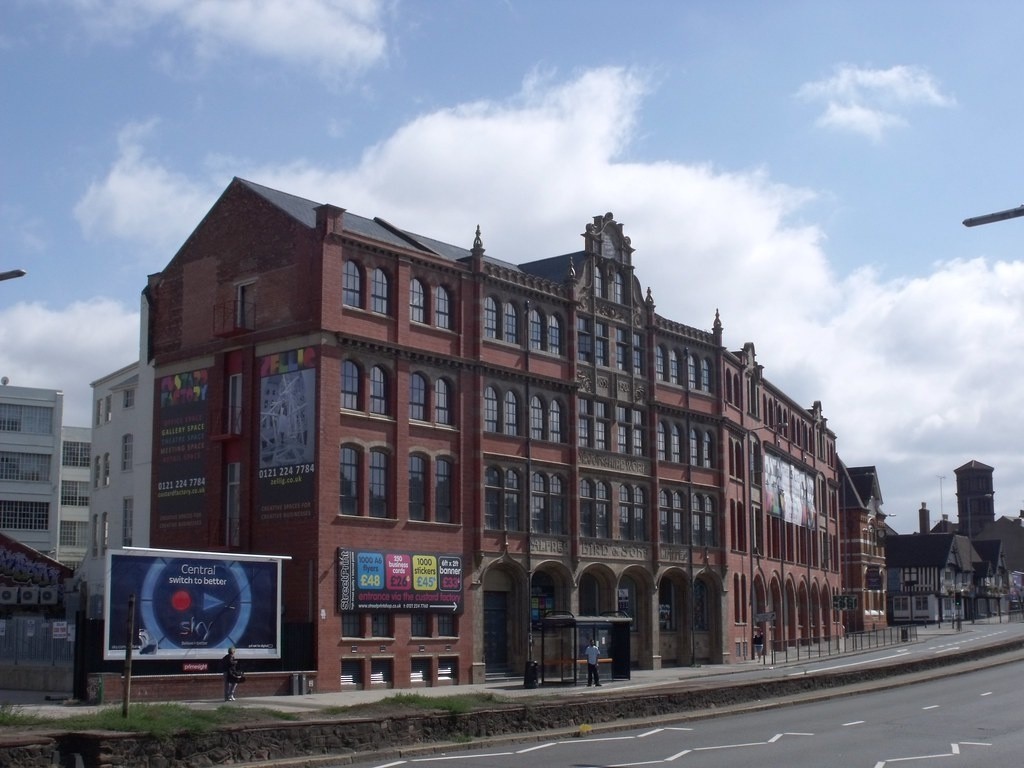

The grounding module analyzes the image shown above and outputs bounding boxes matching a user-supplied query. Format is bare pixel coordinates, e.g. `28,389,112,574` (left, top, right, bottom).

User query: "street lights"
747,422,789,660
967,494,992,586
936,473,945,532
842,472,876,639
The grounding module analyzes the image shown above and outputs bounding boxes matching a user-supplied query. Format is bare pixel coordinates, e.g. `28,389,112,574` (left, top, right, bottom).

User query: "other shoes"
595,683,602,686
228,695,235,701
225,697,232,701
587,683,591,687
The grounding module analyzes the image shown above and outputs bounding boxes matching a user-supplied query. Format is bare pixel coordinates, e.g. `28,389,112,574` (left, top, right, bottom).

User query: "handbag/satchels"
226,670,245,684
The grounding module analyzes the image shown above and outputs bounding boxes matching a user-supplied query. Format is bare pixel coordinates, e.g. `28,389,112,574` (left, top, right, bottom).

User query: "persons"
219,647,238,702
584,639,602,687
752,628,764,663
